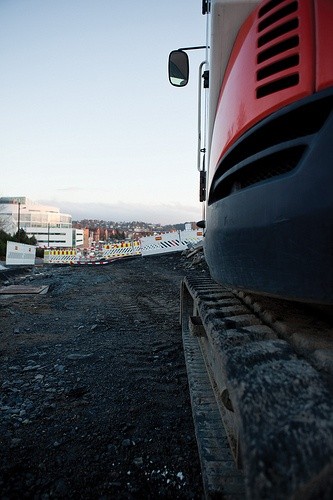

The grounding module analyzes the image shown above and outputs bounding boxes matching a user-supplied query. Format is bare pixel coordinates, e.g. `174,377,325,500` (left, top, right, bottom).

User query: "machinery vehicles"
177,0,333,500
